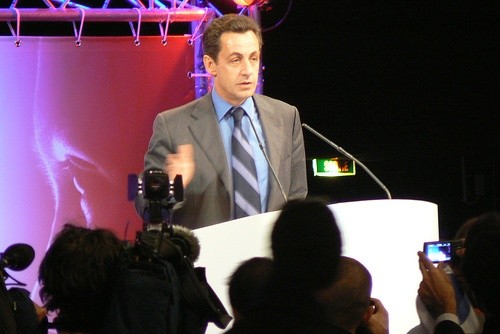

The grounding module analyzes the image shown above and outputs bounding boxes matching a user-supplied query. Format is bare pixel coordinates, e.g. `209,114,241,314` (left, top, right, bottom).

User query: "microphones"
250,117,288,205
302,124,391,199
0,242,36,271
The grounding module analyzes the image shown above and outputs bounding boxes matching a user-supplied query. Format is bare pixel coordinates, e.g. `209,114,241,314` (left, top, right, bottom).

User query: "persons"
135,13,308,231
224,199,389,334
39,223,180,334
408,212,500,334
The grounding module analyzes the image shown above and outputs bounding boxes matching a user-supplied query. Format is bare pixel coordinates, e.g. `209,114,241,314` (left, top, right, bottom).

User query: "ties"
226,107,262,219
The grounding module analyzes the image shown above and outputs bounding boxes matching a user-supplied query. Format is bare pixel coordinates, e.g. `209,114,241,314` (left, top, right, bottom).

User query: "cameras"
423,241,462,263
126,166,183,238
